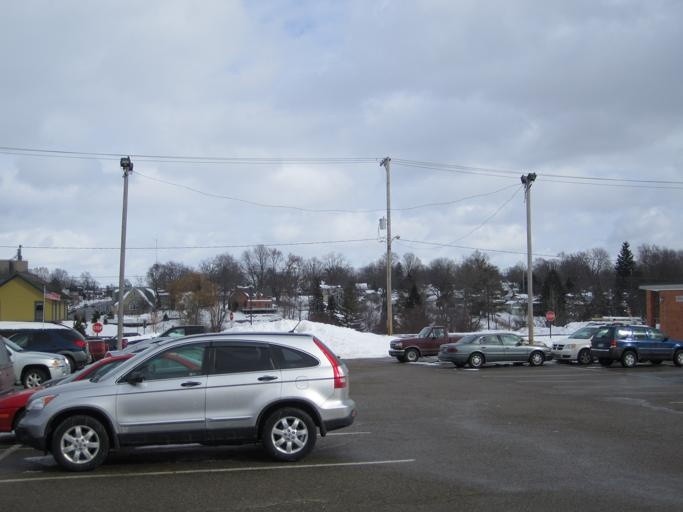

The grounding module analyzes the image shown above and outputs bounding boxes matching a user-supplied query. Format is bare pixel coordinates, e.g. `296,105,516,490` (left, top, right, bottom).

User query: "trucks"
385,324,464,363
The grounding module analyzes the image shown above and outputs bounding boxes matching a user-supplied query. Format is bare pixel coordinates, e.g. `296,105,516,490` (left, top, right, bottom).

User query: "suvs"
438,315,683,372
10,329,360,472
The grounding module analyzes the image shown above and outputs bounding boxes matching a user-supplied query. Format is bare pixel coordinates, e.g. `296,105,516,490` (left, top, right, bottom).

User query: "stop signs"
545,310,556,322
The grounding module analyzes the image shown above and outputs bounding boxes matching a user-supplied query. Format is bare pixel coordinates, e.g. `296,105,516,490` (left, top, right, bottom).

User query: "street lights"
520,173,538,346
113,156,135,351
385,235,402,336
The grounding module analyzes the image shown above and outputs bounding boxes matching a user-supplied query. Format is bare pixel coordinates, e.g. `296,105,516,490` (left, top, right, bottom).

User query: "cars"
0,320,212,433
